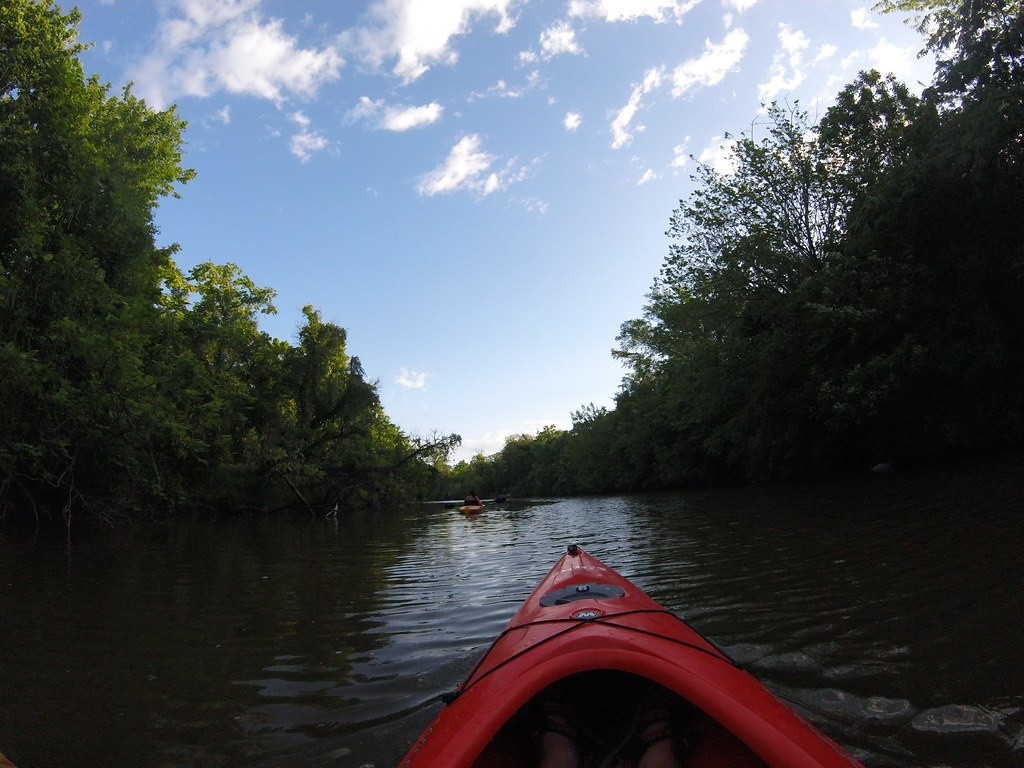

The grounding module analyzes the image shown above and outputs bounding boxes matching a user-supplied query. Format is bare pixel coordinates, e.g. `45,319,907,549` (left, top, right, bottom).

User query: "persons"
464,488,479,505
526,682,685,768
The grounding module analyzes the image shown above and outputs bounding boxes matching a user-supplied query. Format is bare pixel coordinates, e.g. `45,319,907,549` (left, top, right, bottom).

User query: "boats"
489,502,509,508
460,505,484,514
